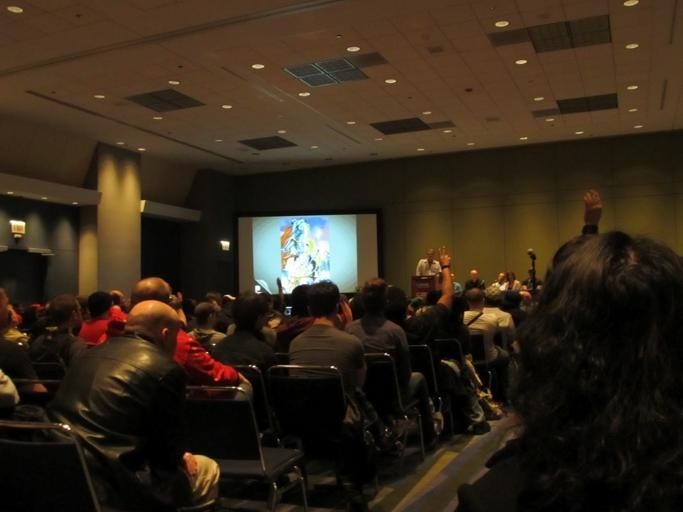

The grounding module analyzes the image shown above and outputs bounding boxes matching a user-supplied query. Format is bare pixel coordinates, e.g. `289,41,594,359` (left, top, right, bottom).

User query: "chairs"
1,332,513,512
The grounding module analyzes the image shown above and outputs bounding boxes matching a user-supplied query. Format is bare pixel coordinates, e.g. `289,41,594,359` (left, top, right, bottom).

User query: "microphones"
527,248,536,261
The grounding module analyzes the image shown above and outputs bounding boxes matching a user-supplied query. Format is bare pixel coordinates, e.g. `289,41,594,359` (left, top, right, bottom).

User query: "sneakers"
469,408,503,436
